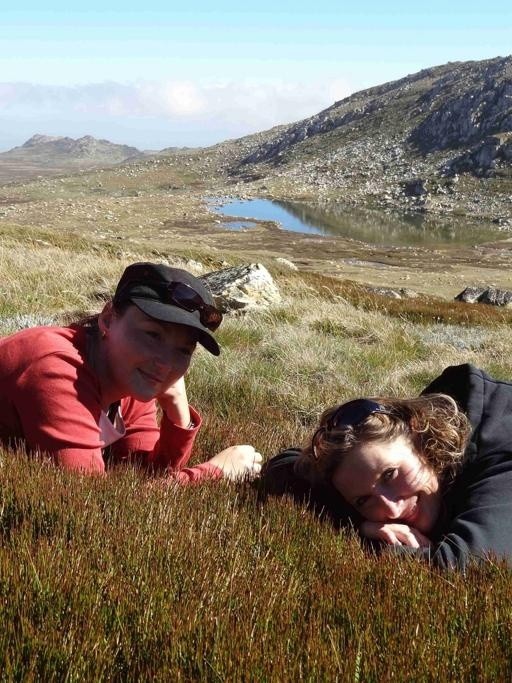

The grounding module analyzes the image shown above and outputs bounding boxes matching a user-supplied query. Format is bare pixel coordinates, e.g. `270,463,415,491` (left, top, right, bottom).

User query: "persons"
262,365,512,570
0,262,262,487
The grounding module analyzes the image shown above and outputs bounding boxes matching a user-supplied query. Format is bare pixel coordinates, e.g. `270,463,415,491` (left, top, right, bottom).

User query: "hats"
115,262,220,356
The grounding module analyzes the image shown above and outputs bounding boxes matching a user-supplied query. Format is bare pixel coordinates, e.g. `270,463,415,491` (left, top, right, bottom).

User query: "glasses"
312,399,389,464
167,281,223,333
